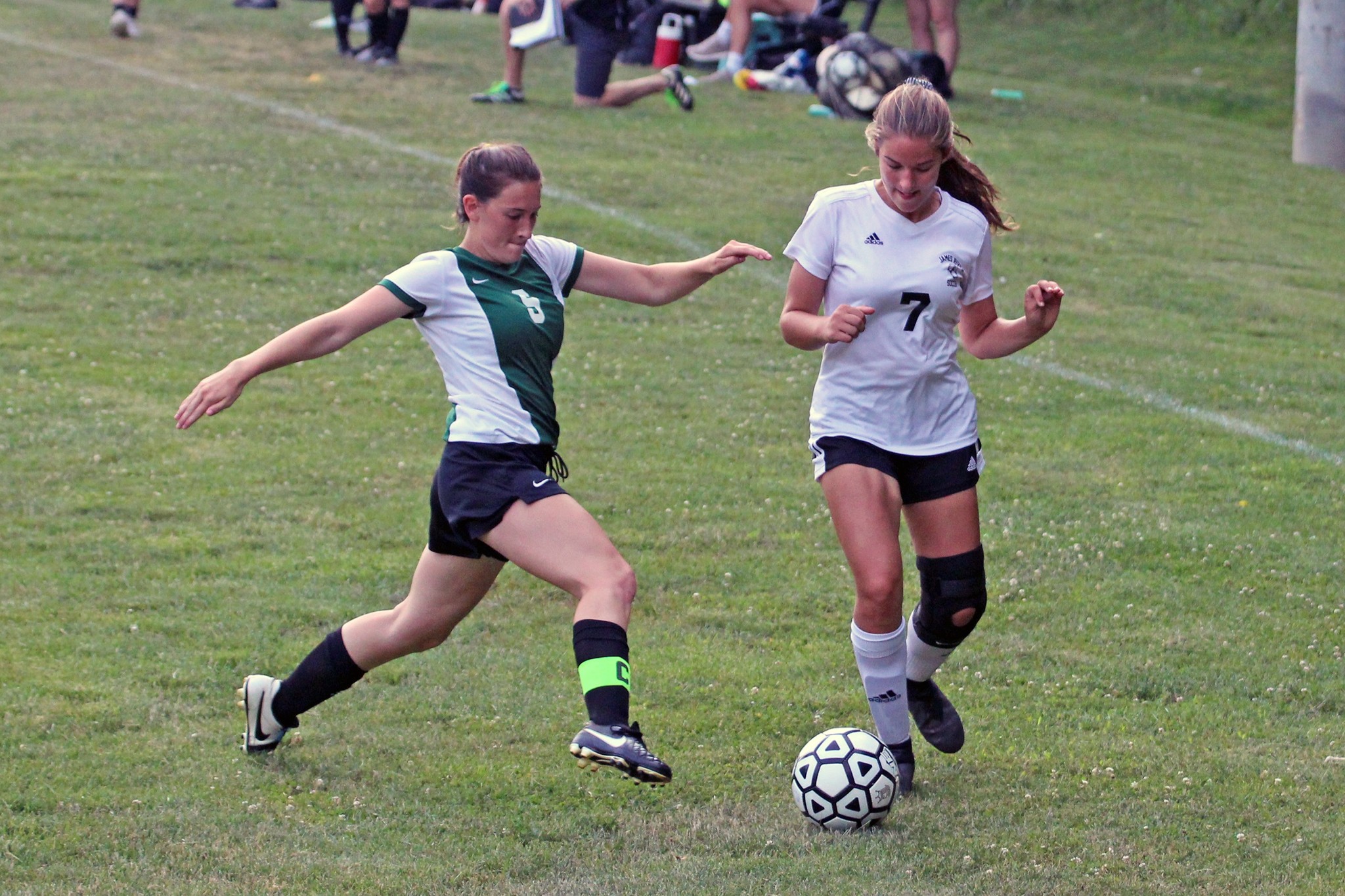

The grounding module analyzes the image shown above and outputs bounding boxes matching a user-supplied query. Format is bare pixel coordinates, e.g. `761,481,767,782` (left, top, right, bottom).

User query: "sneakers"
570,721,672,788
237,674,299,757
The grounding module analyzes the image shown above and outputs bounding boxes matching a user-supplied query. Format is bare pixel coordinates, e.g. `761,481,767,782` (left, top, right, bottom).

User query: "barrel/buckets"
651,12,685,70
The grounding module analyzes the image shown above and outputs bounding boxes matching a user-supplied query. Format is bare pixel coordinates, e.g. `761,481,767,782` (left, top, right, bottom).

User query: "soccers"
790,727,901,834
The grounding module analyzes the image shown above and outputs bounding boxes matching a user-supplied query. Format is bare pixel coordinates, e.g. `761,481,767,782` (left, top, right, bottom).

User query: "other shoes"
356,46,399,66
687,33,731,63
469,81,525,103
110,11,139,38
888,737,915,794
235,0,278,9
660,65,694,111
339,40,369,60
907,677,965,754
701,67,735,84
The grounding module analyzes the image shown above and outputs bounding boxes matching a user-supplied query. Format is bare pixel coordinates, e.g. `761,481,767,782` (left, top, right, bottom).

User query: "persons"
683,0,818,86
329,0,370,60
468,0,695,113
111,1,139,39
779,76,1064,796
358,0,409,65
172,143,770,785
900,0,959,99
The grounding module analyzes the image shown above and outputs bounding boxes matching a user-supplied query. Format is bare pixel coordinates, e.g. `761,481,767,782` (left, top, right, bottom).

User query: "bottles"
807,103,836,118
991,88,1024,98
